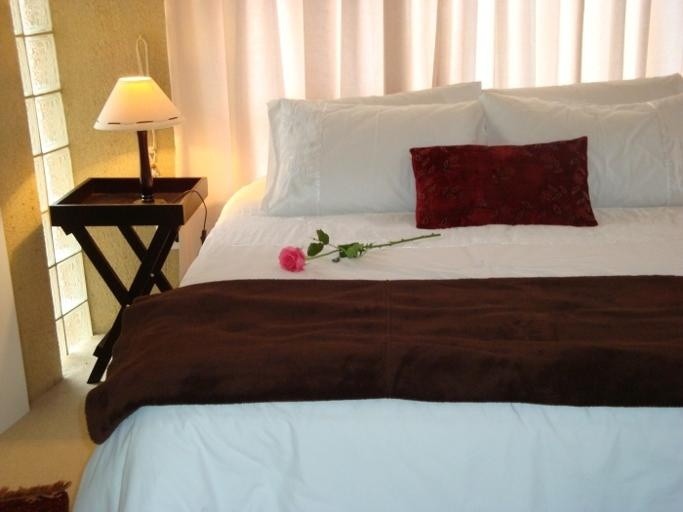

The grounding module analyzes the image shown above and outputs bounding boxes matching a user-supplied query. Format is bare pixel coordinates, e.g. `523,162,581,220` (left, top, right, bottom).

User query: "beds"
64,170,681,512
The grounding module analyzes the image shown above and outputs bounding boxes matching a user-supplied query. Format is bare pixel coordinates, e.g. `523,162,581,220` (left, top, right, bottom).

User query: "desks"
44,176,210,384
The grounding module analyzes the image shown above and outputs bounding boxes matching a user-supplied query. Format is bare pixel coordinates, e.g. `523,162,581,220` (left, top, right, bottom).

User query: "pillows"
263,91,484,223
484,73,682,106
482,90,682,212
260,79,486,216
408,135,601,230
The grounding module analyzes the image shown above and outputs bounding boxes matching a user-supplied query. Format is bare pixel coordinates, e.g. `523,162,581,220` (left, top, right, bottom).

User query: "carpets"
0,473,73,510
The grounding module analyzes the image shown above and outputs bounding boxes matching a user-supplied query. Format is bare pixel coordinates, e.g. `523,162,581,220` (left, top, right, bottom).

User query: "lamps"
91,74,189,205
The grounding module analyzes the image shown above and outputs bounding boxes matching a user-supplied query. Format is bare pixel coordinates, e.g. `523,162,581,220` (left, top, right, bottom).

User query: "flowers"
276,224,442,272
